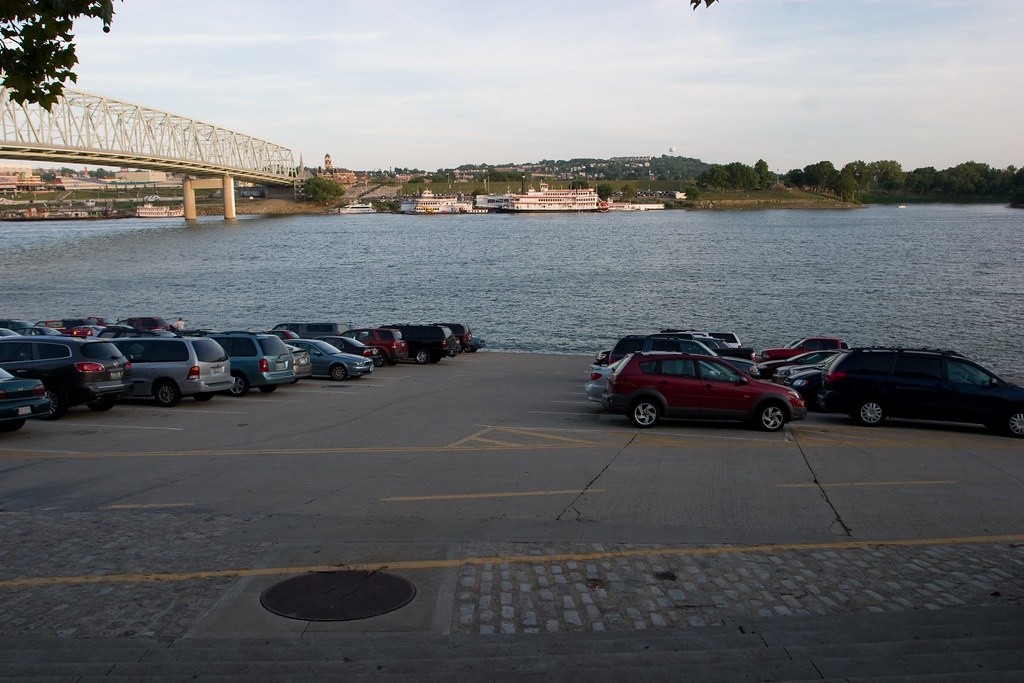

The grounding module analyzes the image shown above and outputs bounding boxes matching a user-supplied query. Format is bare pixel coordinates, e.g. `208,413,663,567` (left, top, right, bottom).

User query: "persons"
175,317,185,332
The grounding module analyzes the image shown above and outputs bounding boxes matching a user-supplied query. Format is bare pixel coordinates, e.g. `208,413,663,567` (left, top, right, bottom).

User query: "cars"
0,367,52,433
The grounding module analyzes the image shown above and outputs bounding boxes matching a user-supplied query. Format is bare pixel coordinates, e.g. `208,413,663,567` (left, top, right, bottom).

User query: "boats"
410,188,610,214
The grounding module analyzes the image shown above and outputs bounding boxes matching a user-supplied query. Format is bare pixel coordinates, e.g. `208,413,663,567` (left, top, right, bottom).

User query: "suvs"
817,347,1024,438
588,328,855,446
0,315,485,419
